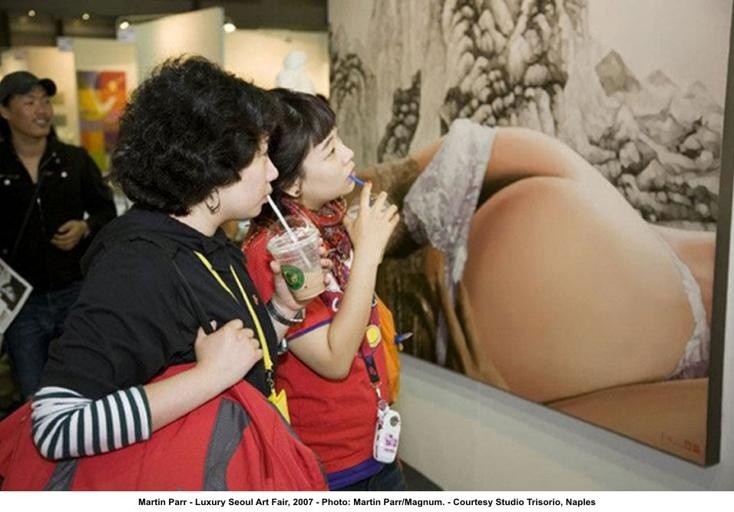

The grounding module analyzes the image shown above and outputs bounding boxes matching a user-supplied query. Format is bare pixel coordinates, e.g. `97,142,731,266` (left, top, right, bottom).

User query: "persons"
0,71,116,406
240,87,408,490
344,118,717,404
30,56,335,462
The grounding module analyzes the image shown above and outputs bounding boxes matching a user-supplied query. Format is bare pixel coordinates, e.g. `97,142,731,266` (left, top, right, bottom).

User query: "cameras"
371,406,403,465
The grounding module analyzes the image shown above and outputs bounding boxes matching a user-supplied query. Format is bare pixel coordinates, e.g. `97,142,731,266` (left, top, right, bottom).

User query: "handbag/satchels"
0,363,331,491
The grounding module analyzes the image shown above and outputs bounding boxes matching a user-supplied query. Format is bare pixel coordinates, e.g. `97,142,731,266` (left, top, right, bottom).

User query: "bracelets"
264,298,306,327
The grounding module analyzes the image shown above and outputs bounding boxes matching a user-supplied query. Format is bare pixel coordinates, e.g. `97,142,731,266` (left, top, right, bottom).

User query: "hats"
1,71,56,97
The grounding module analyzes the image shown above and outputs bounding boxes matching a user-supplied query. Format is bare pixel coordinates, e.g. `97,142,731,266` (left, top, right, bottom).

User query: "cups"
266,227,328,301
347,195,392,263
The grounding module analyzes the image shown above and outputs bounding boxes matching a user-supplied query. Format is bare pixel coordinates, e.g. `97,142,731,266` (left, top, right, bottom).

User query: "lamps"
223,17,238,33
